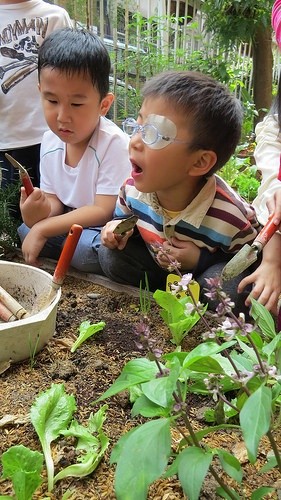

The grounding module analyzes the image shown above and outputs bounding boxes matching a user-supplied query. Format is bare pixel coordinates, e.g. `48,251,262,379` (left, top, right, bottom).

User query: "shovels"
221,216,281,284
6,153,34,196
30,223,83,326
113,214,139,244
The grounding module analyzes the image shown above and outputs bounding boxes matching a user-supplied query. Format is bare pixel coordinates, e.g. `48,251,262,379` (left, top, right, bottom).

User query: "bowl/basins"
0,260,62,363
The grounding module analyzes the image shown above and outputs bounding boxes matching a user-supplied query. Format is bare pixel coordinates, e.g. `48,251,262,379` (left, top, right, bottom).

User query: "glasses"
121,117,213,152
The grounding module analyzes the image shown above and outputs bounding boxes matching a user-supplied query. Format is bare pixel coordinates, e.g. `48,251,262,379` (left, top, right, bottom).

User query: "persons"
0,0,74,247
98,70,262,320
17,28,131,273
237,0,281,320
